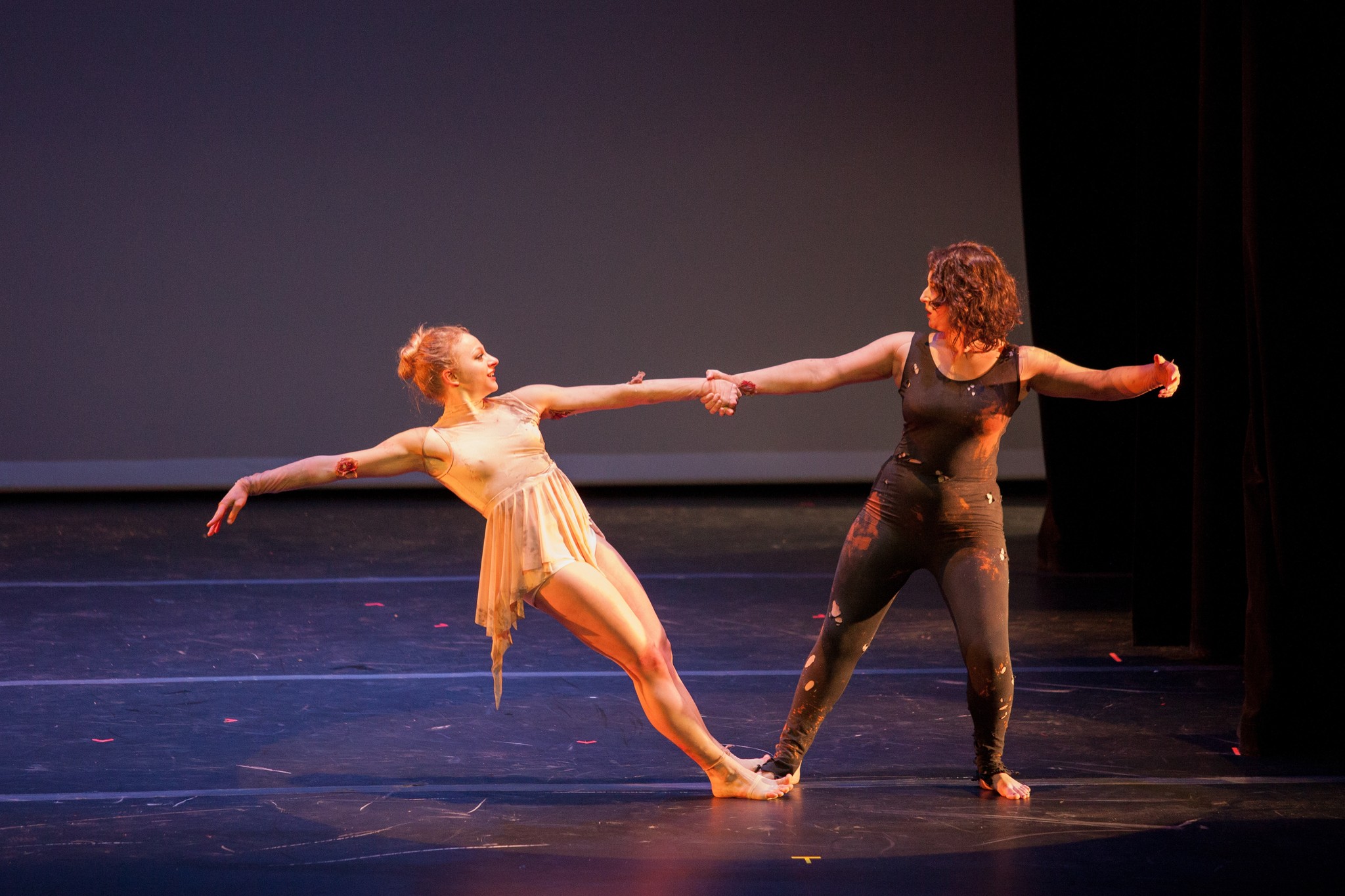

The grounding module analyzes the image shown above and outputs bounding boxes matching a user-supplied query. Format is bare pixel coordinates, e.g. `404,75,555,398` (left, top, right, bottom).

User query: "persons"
206,321,795,799
701,241,1181,802
